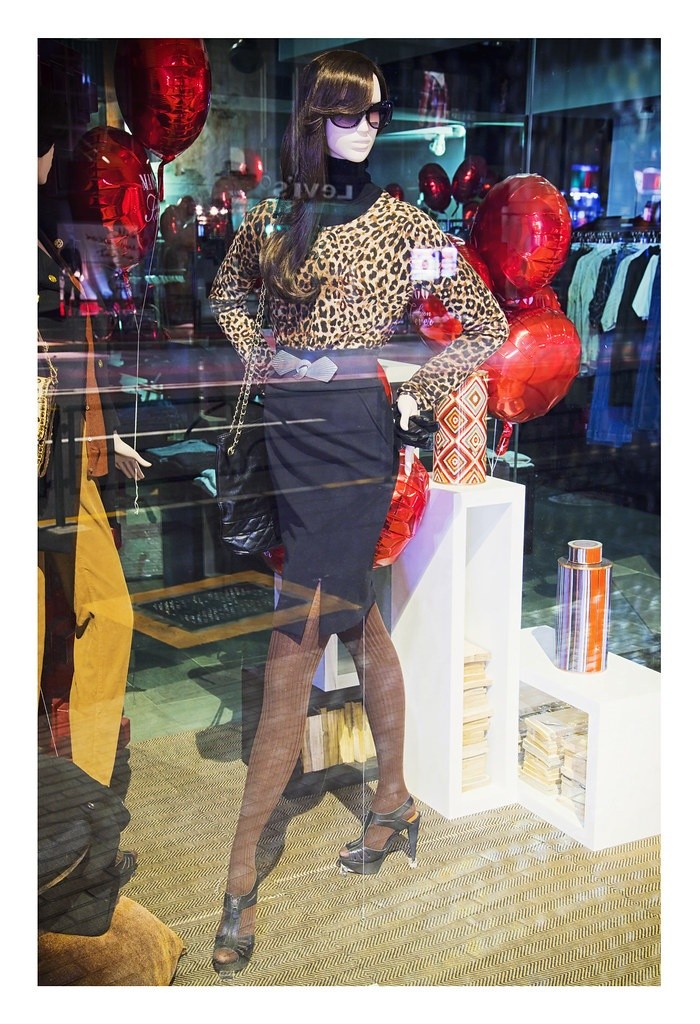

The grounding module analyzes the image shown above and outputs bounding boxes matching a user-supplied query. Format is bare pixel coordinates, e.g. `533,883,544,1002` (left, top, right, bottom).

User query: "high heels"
335,797,422,876
212,878,263,974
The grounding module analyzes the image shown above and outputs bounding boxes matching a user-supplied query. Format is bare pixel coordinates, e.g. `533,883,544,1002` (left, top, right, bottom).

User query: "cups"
434,369,489,486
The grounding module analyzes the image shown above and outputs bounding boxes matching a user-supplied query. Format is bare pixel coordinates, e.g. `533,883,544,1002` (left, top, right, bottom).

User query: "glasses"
327,98,394,130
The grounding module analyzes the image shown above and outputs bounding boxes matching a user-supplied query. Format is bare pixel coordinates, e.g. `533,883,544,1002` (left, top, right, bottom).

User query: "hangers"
568,230,661,250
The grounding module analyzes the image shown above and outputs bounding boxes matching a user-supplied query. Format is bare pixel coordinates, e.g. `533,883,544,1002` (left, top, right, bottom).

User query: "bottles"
553,542,611,674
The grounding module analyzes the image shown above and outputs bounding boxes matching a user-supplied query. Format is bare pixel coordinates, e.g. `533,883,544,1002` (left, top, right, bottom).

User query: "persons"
39,112,152,833
208,50,510,973
60,239,82,316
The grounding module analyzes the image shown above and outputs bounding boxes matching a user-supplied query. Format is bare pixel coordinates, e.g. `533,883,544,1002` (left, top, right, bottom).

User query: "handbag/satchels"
215,415,281,558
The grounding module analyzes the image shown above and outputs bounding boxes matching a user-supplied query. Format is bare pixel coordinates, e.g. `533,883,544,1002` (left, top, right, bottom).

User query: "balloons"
462,200,479,228
67,125,158,273
384,183,404,201
478,173,500,199
413,174,582,421
452,154,489,203
418,163,452,212
212,148,264,210
114,38,212,164
160,196,196,247
